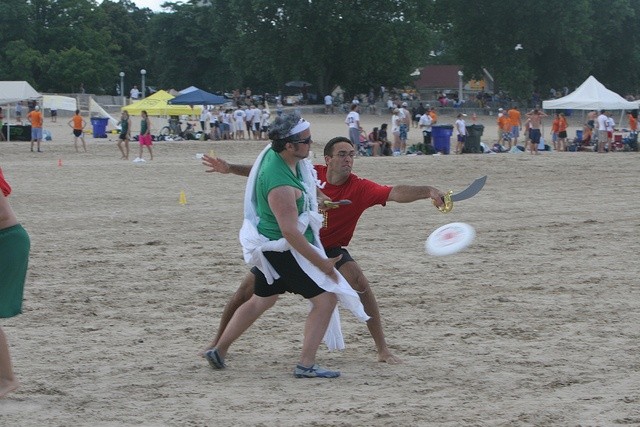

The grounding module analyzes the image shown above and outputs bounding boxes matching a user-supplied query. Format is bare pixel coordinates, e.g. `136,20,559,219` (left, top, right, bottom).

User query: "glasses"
291,135,311,143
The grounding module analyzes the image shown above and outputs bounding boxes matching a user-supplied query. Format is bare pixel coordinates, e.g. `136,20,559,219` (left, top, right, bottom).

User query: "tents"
121,88,202,139
542,75,640,149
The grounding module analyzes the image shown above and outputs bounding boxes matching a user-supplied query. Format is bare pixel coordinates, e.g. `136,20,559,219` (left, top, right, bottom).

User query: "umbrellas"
0,81,43,141
178,85,199,94
167,89,225,140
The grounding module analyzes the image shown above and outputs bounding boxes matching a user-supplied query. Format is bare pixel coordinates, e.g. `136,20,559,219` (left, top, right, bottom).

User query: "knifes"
433,175,487,214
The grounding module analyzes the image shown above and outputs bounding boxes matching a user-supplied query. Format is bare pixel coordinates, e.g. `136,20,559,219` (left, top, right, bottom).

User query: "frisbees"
424,222,476,257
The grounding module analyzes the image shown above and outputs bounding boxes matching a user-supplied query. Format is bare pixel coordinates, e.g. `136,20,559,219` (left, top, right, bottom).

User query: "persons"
411,107,417,125
497,110,506,145
507,106,523,146
587,110,596,124
130,84,139,100
0,166,31,393
525,109,548,155
138,110,153,160
201,135,445,363
455,113,469,153
200,106,271,141
204,109,343,378
391,108,401,152
67,109,87,153
116,110,131,160
597,109,609,153
437,86,492,109
377,123,393,156
366,126,382,156
51,109,58,122
428,108,438,123
324,85,418,114
501,111,512,151
345,104,363,149
551,113,560,150
607,114,615,151
418,103,426,122
627,110,637,132
558,114,568,150
524,117,532,149
228,87,285,108
419,108,436,146
26,104,43,152
398,102,412,151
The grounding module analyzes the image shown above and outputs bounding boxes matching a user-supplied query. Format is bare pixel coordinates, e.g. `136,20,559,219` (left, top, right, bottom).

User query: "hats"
401,102,407,106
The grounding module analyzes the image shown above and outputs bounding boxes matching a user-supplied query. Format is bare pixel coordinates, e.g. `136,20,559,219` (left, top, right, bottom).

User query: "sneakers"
206,350,225,368
295,363,340,377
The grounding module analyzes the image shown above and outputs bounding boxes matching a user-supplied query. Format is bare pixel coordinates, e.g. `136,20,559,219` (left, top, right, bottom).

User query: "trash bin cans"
169,115,179,132
464,124,484,153
433,125,453,154
